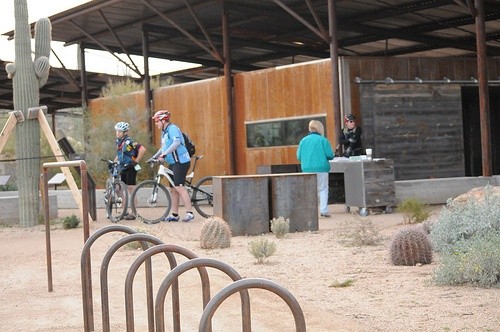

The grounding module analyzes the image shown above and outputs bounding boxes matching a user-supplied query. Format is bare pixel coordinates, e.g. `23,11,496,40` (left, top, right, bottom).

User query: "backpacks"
181,132,195,158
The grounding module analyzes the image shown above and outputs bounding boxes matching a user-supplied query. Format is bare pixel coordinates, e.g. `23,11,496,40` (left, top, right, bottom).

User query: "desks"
328,160,397,215
211,173,318,234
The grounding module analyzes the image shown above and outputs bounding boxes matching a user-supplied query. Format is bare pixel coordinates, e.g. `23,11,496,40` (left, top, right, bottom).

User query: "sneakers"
162,213,180,221
115,211,129,218
123,211,136,220
182,211,194,222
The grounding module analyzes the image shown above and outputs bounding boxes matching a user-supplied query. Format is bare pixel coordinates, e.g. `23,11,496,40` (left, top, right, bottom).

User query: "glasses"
350,120,356,123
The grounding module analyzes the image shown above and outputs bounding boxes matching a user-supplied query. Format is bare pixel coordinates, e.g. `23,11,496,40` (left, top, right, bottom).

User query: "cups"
366,149,372,160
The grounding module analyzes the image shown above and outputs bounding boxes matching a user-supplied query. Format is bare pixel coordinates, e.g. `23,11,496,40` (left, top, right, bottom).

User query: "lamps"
414,76,423,84
470,76,478,83
386,76,394,83
354,76,363,84
442,75,451,83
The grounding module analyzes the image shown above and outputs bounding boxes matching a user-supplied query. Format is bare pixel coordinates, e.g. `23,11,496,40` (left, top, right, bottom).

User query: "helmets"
151,110,171,122
344,113,357,123
114,121,130,132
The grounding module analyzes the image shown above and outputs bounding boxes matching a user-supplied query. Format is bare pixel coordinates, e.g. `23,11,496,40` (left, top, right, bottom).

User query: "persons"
297,120,335,216
113,122,146,220
152,110,195,222
334,114,364,158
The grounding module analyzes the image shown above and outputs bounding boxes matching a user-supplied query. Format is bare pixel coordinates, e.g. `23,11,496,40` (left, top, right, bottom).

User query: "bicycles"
100,158,132,223
130,155,215,224
